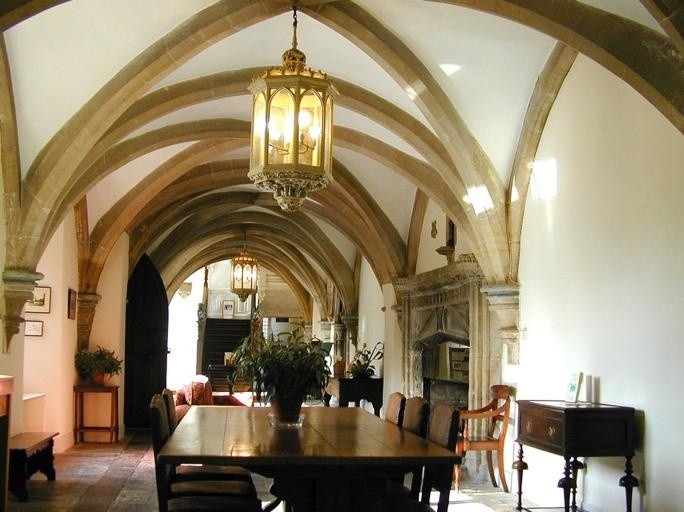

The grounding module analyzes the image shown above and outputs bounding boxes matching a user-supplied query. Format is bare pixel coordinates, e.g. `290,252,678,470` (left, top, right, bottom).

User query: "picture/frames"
221,300,235,319
24,285,52,314
24,319,43,337
68,289,77,320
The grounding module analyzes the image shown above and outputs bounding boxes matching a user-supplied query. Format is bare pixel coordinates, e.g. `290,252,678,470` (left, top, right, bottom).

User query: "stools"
8,432,60,503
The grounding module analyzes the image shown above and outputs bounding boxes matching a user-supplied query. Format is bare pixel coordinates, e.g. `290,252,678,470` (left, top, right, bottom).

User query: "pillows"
184,375,214,405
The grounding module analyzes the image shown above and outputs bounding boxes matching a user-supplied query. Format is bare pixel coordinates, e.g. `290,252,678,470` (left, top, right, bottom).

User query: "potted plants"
196,302,207,327
227,330,330,429
74,347,123,387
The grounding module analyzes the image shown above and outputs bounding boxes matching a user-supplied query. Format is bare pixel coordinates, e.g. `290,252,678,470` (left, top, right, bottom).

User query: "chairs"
386,393,460,512
147,389,262,512
454,385,517,493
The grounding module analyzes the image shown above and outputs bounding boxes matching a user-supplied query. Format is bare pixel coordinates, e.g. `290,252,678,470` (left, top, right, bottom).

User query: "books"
563,370,583,403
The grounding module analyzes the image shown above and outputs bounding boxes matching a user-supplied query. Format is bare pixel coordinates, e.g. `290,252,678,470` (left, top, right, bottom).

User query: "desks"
338,378,383,417
72,385,119,444
159,406,454,512
512,400,639,512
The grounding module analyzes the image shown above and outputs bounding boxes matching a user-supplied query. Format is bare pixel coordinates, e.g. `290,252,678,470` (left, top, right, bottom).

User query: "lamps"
231,231,258,303
247,0,341,212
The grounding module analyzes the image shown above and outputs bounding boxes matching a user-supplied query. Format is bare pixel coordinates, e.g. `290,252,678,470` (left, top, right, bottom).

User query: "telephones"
566,372,583,405
